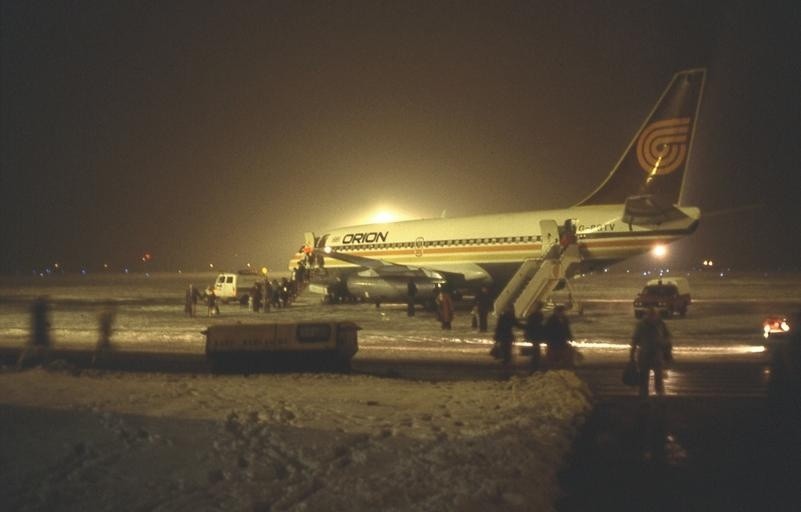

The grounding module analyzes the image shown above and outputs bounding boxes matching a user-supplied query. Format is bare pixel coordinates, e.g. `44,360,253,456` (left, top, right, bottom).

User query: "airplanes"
288,67,710,329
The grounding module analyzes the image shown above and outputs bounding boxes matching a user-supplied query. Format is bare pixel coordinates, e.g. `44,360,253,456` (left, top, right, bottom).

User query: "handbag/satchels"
622,360,640,386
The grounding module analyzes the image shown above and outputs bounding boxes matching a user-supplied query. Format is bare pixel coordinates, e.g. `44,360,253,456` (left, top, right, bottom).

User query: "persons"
249,252,314,314
403,278,418,316
202,284,219,317
628,304,672,400
490,302,526,367
544,303,574,366
92,304,117,353
473,284,492,332
433,290,455,330
27,293,53,346
184,282,201,318
523,301,550,367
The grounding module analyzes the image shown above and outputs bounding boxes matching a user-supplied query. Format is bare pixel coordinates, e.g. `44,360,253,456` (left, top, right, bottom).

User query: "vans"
213,272,275,305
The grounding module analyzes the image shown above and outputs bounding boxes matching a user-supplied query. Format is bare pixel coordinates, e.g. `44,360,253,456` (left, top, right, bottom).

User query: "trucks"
633,276,694,321
